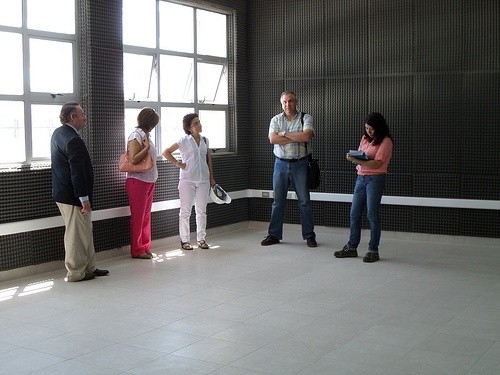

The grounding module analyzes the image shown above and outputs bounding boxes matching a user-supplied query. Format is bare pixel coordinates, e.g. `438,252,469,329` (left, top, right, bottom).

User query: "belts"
277,153,310,163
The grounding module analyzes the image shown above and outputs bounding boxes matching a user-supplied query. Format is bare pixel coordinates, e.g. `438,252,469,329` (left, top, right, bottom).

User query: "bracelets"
176,161,182,167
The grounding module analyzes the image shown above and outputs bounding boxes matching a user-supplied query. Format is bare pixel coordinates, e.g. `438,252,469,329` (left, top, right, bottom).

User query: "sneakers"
307,238,316,247
363,252,380,263
261,235,280,246
334,245,358,257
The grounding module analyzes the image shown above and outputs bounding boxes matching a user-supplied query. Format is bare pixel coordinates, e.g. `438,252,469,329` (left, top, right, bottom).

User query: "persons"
126,108,160,259
260,90,317,248
51,102,109,282
334,112,394,263
162,112,216,250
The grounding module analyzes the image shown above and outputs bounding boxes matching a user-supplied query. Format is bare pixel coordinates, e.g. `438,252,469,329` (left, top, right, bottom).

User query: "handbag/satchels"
119,132,154,172
307,153,320,189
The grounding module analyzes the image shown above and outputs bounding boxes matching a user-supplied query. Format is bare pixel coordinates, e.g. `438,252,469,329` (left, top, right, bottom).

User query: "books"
349,150,367,159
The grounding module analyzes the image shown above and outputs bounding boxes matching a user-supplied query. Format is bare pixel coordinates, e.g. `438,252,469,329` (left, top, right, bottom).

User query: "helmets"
210,184,231,205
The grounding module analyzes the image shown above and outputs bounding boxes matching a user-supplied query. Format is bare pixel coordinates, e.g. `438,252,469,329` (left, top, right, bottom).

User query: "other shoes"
135,251,154,259
79,273,96,282
90,269,109,277
197,240,209,248
181,241,193,249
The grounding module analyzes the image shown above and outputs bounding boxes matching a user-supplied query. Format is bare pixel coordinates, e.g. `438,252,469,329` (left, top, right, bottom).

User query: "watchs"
282,130,288,136
